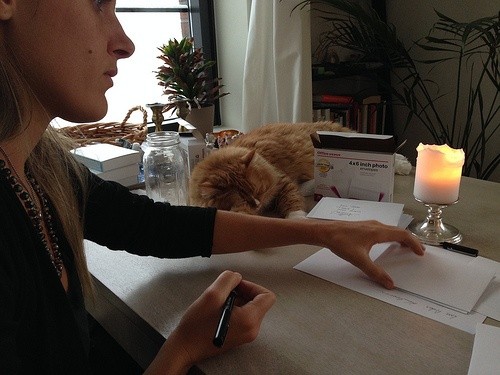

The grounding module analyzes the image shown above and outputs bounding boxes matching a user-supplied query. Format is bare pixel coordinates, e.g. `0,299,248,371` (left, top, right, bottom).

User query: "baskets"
55,105,148,149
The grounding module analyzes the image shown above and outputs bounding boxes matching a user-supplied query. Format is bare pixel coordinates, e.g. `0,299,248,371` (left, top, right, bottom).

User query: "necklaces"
0,159,62,278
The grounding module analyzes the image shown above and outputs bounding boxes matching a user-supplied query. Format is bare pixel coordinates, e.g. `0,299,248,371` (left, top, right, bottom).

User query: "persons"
0,0,426,375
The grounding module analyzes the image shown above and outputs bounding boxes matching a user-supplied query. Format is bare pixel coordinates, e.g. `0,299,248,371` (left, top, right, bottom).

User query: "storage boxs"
177,116,208,177
308,130,396,202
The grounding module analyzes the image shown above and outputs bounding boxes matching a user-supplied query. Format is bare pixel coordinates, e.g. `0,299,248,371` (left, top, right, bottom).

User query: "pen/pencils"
212,293,235,348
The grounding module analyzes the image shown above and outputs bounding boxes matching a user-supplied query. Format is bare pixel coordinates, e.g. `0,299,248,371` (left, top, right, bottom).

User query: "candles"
413,142,465,204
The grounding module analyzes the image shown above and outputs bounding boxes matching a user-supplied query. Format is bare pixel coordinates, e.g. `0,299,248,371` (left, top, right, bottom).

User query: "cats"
189,120,413,220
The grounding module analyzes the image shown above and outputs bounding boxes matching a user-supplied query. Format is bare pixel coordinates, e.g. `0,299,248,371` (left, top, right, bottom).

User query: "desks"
83,125,500,375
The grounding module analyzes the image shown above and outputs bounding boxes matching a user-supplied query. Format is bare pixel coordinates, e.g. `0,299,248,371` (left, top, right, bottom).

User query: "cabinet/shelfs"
311,0,396,135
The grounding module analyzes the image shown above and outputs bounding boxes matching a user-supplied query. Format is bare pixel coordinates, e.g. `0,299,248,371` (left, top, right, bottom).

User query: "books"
70,144,139,186
312,95,392,136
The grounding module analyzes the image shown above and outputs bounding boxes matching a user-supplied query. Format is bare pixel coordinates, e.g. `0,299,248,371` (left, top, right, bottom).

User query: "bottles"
142,131,190,206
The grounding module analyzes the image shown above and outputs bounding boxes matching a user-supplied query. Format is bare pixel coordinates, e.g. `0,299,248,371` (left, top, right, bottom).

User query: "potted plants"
152,35,231,139
